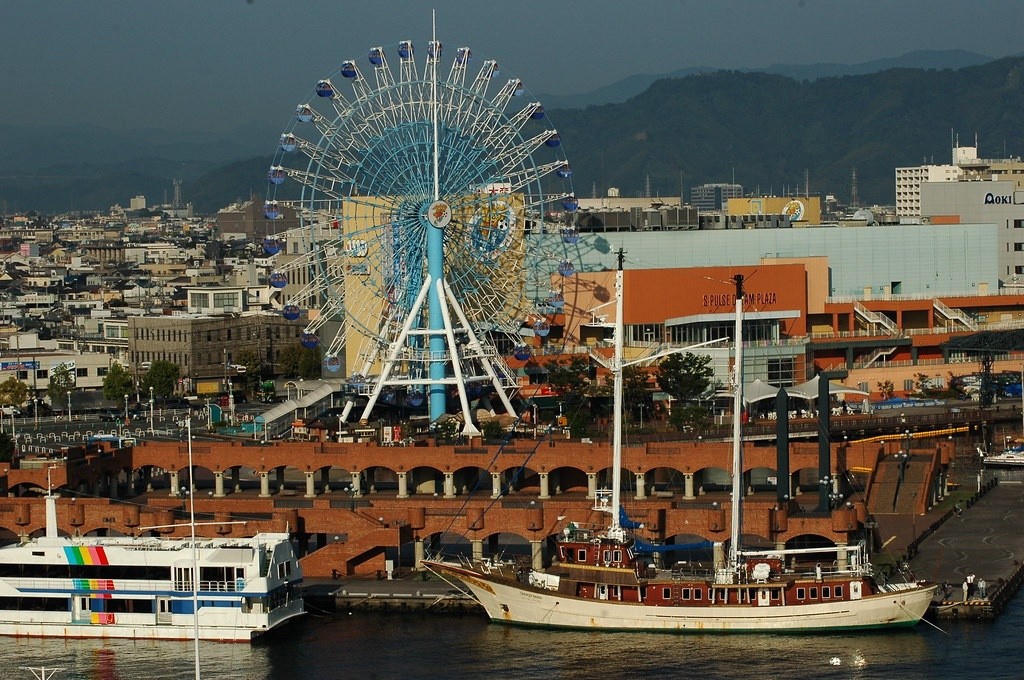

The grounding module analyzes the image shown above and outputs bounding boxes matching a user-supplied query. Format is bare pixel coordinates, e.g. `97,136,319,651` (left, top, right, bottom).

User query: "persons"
962,578,969,601
954,504,962,517
978,578,986,598
842,399,847,416
942,578,952,600
967,573,975,596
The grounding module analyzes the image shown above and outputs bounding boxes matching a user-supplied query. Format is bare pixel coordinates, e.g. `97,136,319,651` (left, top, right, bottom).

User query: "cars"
0,403,21,418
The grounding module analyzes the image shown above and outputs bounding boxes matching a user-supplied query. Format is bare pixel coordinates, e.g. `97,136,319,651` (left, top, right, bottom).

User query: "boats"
0,490,309,645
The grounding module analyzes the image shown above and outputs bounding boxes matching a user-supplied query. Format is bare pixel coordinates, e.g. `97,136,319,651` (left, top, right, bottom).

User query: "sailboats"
416,244,942,634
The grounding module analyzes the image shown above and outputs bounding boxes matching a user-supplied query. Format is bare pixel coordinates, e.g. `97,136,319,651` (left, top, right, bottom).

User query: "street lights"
203,397,212,430
638,402,645,429
33,399,38,430
899,430,914,458
377,417,385,444
818,475,834,513
148,386,154,399
828,491,844,509
335,414,344,438
532,403,538,439
66,391,72,423
343,482,359,513
175,487,191,518
149,399,155,430
893,450,907,481
9,405,17,438
124,394,129,426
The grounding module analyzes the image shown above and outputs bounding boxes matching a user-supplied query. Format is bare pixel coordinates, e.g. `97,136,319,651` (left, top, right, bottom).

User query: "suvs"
98,407,123,423
